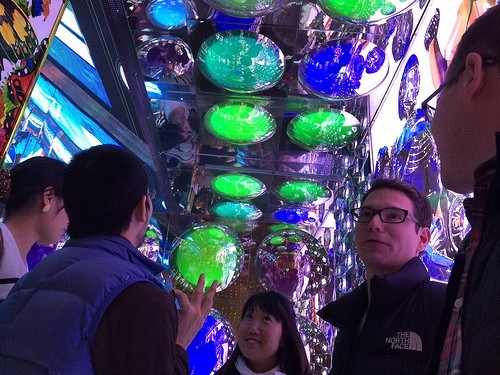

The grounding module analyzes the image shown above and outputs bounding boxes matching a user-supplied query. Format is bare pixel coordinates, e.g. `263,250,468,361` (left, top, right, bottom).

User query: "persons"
316,178,449,375
421,4,500,375
213,290,313,375
0,156,69,306
0,144,218,375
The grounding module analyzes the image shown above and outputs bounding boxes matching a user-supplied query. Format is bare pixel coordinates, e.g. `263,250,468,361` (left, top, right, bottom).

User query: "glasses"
421,57,499,123
144,186,158,199
350,206,422,227
